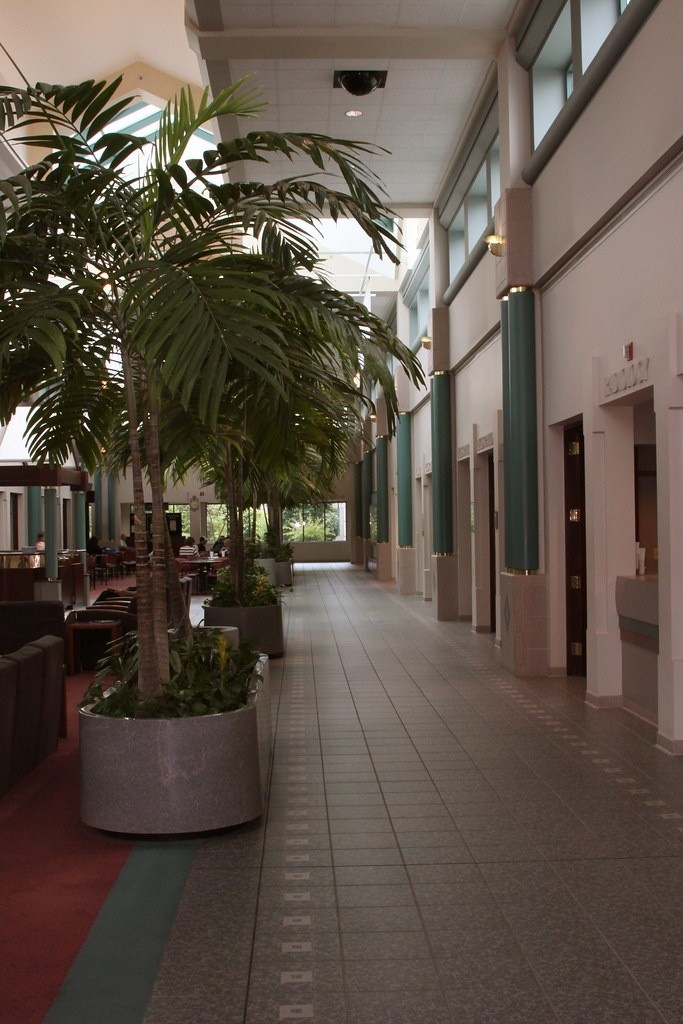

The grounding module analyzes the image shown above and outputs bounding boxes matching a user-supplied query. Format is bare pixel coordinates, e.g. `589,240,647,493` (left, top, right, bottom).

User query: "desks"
176,555,229,592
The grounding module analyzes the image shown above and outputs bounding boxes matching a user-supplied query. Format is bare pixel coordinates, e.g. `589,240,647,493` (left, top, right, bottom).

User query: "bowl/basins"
22,546,37,553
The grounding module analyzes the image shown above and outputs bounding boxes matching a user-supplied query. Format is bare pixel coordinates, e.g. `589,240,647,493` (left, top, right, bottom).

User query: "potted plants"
0,69,426,839
252,518,276,588
102,247,378,655
276,537,298,587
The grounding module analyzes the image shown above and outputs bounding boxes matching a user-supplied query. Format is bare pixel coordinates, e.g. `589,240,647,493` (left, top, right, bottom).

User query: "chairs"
86,545,229,594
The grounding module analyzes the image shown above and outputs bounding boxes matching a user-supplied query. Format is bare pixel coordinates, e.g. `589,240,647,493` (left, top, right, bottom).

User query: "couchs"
0,600,64,654
76,577,192,670
0,635,64,799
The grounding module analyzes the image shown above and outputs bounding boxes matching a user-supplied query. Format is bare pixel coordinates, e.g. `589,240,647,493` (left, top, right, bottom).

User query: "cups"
222,551,225,557
210,552,214,560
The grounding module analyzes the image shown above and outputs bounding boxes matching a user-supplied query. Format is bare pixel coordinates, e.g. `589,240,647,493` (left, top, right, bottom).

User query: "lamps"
420,337,431,348
367,412,377,422
483,234,498,254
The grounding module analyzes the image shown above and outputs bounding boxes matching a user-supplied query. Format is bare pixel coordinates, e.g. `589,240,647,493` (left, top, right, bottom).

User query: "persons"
86,535,107,566
179,536,200,557
211,535,230,557
197,536,208,552
118,533,128,548
34,531,46,551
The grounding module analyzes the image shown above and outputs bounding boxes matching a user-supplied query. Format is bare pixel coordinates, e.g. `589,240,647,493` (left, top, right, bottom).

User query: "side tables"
67,620,122,676
